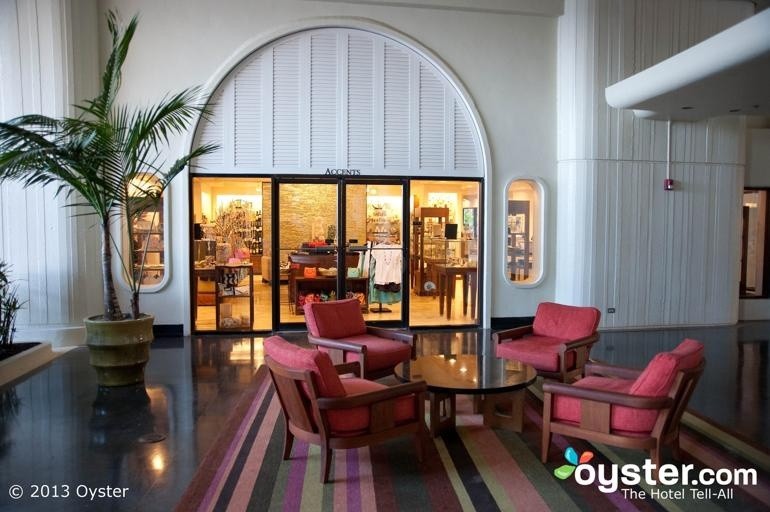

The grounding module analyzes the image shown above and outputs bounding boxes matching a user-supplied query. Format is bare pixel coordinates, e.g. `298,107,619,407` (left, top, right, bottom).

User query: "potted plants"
1,7,225,388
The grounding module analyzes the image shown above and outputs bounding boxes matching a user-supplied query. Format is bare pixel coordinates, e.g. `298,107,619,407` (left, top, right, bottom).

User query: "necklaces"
382,243,393,266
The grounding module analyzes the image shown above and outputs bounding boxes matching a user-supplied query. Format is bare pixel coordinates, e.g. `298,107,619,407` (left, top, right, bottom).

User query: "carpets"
171,341,770,511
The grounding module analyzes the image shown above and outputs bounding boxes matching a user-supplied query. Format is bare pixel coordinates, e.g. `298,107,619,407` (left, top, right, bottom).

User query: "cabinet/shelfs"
286,249,373,315
413,205,454,295
214,262,255,334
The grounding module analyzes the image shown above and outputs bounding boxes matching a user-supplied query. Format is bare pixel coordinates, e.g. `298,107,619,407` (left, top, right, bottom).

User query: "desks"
433,263,479,320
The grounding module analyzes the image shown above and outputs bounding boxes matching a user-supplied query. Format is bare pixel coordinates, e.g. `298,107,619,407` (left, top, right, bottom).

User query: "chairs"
264,281,422,485
490,303,707,486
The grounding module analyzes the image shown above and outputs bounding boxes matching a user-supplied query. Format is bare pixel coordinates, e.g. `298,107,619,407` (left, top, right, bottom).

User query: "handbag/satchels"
347,267,360,278
304,266,316,279
217,272,250,297
296,290,366,310
234,248,250,260
